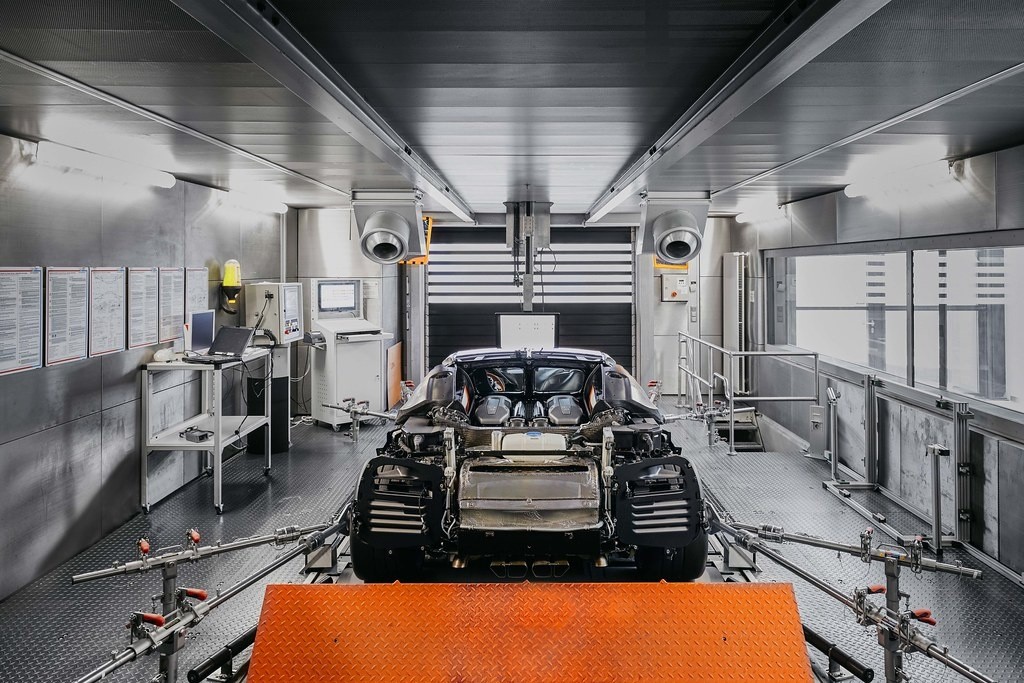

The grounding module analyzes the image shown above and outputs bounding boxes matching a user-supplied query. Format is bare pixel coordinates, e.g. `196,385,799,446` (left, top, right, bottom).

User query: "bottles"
222,259,242,303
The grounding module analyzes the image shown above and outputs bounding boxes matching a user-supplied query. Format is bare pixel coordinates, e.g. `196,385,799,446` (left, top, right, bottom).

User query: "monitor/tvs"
184,309,216,355
319,283,355,312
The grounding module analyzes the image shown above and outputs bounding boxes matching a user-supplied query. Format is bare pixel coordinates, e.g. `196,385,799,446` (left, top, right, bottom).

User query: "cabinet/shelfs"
139,348,273,515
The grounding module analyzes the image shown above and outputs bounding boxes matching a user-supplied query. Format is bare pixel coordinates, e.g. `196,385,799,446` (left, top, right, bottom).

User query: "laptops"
184,326,256,364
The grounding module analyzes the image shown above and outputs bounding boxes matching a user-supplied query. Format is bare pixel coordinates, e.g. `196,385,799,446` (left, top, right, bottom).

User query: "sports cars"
349,346,709,583
